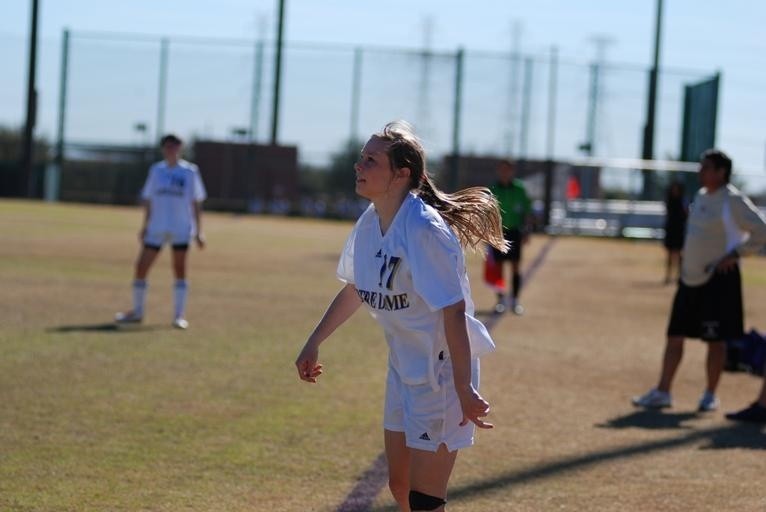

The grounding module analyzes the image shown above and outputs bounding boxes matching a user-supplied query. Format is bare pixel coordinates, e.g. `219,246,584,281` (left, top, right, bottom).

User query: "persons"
295,121,513,512
114,134,208,329
663,181,690,284
631,149,766,411
486,159,534,315
726,374,766,422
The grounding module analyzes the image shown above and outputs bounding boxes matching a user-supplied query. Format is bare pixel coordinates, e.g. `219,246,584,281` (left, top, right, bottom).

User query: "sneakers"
632,389,672,408
700,394,716,411
